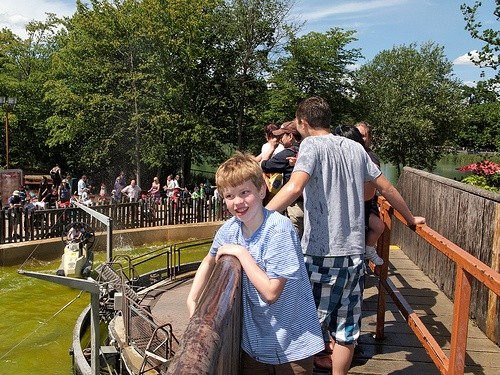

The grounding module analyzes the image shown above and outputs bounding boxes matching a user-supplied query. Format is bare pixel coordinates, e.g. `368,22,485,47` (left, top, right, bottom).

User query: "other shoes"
315,354,332,369
324,342,334,354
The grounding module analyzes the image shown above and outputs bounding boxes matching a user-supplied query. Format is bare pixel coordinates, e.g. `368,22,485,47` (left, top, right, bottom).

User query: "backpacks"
51,168,58,180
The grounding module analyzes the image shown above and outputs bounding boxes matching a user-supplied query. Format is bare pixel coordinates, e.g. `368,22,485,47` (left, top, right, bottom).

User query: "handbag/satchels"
265,172,282,194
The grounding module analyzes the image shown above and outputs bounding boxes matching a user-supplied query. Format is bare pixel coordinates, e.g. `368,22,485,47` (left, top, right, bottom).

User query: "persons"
255,95,425,375
7,163,232,242
186,150,325,375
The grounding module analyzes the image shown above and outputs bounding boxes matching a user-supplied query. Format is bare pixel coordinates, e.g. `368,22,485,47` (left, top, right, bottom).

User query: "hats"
13,190,19,196
272,121,298,136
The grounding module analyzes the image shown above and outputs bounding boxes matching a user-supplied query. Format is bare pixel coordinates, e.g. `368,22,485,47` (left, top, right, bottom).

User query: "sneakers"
365,249,384,265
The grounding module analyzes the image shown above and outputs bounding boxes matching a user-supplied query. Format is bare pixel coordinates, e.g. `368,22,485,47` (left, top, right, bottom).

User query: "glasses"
335,124,344,136
62,182,66,184
153,180,156,182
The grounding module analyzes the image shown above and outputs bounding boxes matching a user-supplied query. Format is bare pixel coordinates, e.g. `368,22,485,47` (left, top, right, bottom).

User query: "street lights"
0,96,19,169
